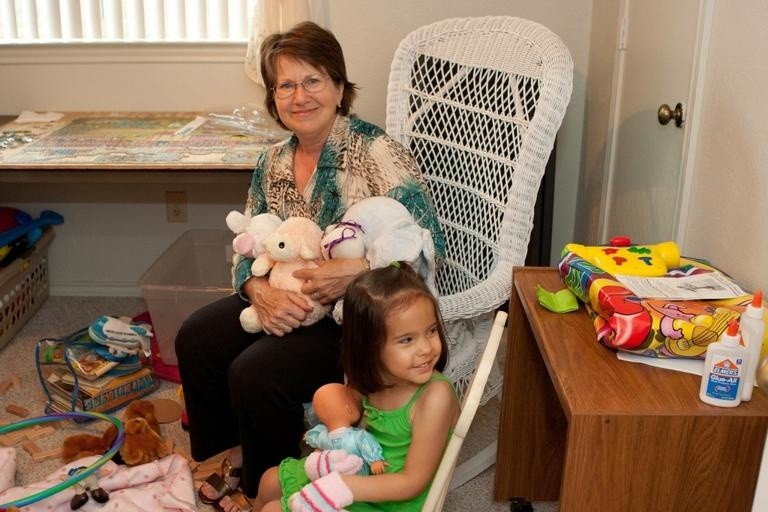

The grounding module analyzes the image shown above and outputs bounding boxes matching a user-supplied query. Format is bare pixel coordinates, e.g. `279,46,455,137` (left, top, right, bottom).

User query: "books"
44,356,150,417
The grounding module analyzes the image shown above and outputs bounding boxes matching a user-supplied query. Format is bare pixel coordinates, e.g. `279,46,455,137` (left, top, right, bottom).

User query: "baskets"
0,224,55,350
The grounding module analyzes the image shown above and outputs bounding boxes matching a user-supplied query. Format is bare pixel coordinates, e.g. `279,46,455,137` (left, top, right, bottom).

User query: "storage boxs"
137,229,237,366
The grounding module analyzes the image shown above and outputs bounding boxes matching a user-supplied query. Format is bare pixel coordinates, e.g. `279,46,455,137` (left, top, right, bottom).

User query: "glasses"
269,74,331,99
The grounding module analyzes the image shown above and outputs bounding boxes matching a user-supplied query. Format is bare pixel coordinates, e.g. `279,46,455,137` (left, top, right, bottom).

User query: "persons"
302,382,391,477
254,260,462,511
171,21,447,511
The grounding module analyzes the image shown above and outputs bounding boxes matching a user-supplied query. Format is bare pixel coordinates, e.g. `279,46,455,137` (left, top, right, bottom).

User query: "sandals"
198,457,253,512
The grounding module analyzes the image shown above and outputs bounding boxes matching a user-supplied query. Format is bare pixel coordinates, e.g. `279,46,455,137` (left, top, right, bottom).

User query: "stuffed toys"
239,215,331,335
87,314,154,362
226,205,282,256
321,194,435,324
64,398,165,467
59,465,112,509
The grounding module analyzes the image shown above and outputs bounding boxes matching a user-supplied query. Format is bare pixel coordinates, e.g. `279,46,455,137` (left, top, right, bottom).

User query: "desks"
492,266,768,512
0,112,290,170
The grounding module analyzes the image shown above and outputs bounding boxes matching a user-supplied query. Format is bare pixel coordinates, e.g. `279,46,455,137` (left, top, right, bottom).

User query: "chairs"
334,15,573,494
421,310,509,512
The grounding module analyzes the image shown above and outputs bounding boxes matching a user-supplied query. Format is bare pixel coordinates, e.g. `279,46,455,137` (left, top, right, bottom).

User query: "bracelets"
359,256,372,270
